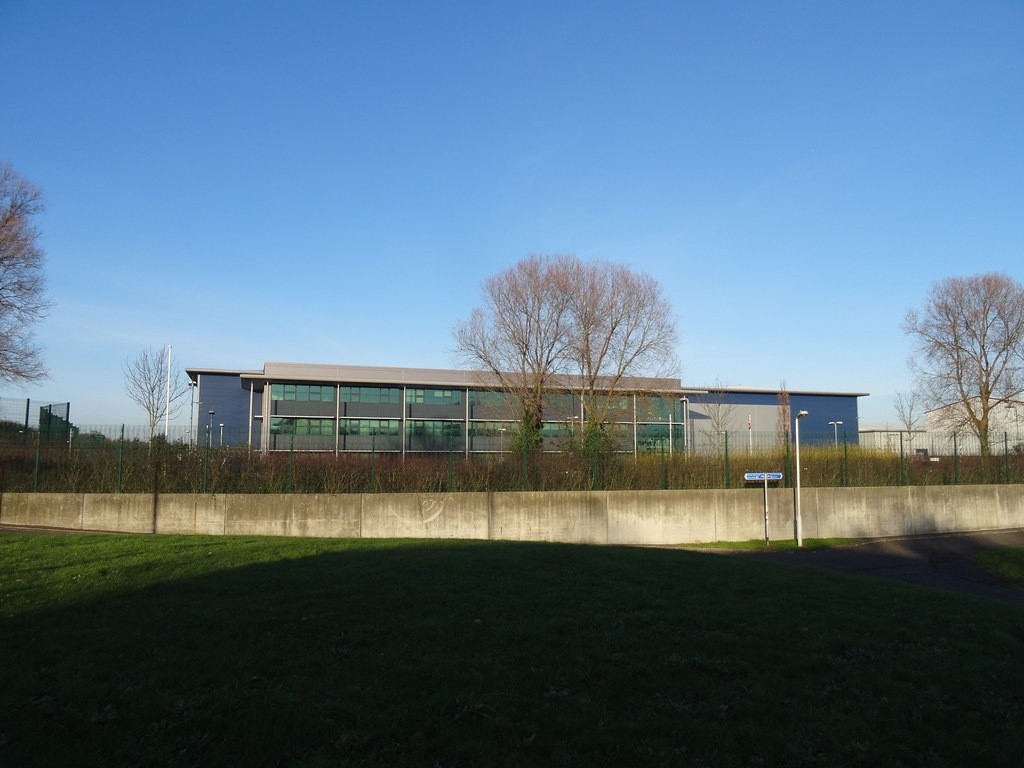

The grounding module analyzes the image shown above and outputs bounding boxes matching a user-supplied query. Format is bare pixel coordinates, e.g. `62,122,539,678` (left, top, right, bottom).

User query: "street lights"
796,410,808,548
567,416,579,440
219,424,225,448
189,381,197,450
208,410,215,449
498,429,506,463
828,421,843,451
679,398,691,461
